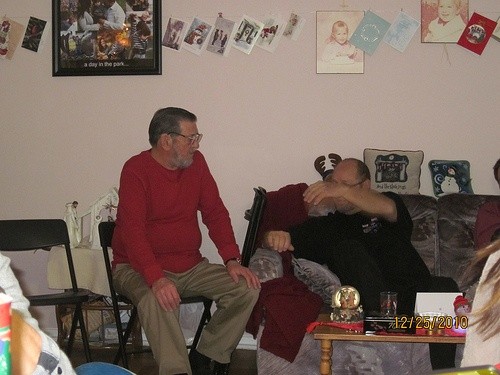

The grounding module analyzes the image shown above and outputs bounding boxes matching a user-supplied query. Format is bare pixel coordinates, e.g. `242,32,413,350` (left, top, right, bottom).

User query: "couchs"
246,192,500,375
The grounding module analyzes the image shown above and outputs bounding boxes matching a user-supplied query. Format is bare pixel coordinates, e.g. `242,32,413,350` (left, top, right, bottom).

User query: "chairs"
0,218,91,362
98,221,213,369
239,185,267,267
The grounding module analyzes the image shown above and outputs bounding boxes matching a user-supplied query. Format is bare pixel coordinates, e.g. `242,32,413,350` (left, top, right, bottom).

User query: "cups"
380,291,397,316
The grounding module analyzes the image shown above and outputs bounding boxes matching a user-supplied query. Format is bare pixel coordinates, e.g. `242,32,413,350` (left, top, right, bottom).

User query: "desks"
315,314,467,375
47,245,144,351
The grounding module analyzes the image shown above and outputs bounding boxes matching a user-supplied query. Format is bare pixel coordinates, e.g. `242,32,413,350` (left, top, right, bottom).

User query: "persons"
325,21,357,60
0,249,77,375
113,107,262,375
76,0,151,61
424,0,467,41
460,158,500,368
266,158,458,371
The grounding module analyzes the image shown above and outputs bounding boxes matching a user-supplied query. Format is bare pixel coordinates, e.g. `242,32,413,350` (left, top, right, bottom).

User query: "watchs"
225,257,241,266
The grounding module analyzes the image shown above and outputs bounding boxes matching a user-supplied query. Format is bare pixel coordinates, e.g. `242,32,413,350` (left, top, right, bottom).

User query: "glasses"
168,131,203,143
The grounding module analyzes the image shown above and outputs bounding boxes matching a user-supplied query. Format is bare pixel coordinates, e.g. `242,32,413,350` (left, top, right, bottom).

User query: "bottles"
453,296,471,322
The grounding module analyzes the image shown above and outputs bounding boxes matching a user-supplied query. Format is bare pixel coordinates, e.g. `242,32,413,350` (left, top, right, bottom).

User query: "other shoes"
189,349,230,375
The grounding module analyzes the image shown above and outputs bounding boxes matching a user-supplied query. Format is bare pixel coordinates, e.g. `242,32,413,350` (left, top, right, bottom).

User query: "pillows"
290,254,338,302
363,149,425,195
429,160,474,196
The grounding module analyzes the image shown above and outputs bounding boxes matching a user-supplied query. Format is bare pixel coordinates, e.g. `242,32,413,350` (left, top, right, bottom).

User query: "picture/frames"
52,0,162,78
